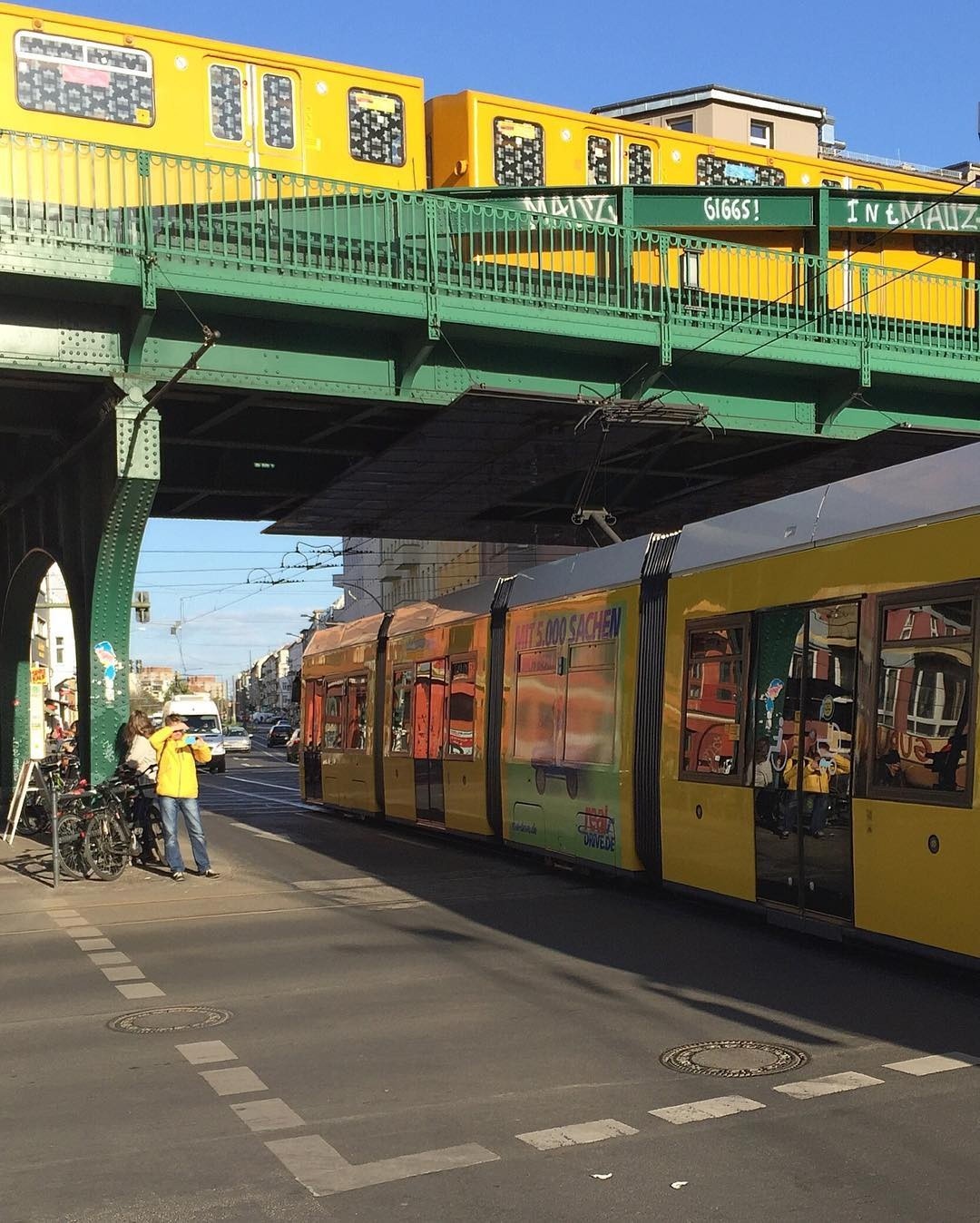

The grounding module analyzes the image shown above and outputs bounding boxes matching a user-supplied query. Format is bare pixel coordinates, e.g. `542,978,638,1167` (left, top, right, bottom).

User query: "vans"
161,694,226,773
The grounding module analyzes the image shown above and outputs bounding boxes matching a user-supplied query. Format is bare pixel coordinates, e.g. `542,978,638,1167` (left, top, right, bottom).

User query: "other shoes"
807,829,826,838
781,830,789,839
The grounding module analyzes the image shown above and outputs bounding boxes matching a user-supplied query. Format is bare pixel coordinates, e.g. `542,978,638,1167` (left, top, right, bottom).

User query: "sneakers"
171,871,185,882
200,869,220,878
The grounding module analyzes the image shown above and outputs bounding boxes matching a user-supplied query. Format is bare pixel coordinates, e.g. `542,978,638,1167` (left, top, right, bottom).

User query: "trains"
0,2,980,330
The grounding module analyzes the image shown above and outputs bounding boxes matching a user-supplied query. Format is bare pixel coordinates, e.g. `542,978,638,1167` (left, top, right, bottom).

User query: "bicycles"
14,753,169,880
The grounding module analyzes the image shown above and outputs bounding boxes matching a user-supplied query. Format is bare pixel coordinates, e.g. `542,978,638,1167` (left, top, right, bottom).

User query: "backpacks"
756,763,768,787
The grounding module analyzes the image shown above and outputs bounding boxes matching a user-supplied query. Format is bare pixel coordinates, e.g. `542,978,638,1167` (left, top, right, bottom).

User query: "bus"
292,440,980,967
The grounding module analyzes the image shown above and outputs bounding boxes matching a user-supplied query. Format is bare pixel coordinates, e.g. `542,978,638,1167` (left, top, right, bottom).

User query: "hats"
885,750,900,764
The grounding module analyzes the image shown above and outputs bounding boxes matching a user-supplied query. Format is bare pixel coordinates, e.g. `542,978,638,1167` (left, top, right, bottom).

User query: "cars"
266,725,293,748
284,729,301,763
222,726,253,753
277,721,290,725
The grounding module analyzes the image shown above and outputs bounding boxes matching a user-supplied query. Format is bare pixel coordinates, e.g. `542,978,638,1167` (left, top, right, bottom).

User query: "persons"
774,741,851,839
876,749,902,785
122,710,165,862
149,715,219,881
